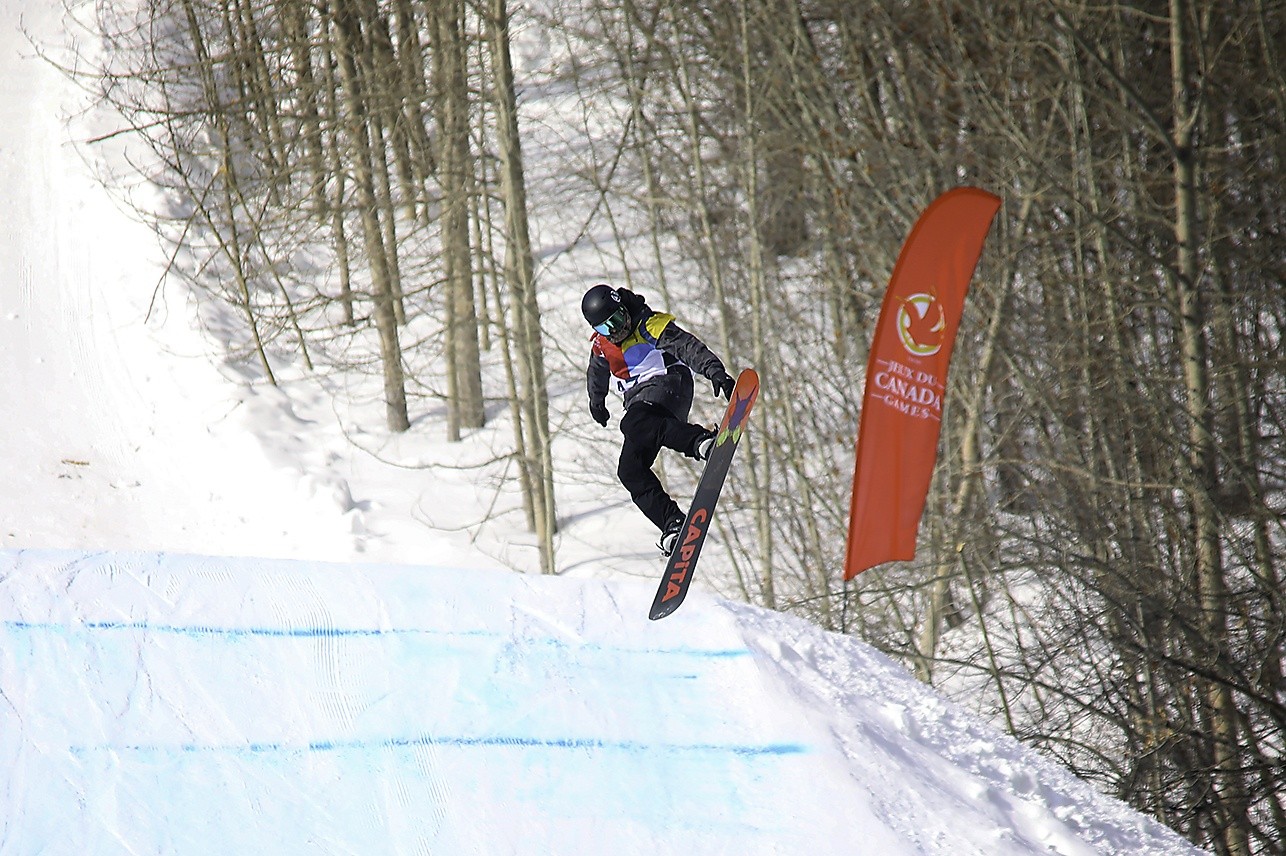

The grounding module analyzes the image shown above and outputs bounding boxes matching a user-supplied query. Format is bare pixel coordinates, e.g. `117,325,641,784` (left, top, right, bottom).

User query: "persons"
581,284,735,557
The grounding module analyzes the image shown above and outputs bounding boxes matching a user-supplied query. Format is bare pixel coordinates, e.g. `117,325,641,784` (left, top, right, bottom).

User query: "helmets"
582,285,623,327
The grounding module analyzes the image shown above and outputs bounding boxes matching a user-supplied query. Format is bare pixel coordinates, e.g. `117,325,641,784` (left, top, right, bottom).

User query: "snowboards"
647,367,760,622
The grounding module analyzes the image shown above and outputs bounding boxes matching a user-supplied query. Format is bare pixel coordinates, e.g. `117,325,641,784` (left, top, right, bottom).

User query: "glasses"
592,305,623,336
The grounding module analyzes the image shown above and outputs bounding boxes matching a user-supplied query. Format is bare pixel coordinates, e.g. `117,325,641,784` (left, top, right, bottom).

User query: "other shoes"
656,517,682,557
693,432,715,461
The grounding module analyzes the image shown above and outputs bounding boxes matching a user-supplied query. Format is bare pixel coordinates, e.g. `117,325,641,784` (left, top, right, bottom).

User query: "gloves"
712,371,735,402
590,401,610,427
